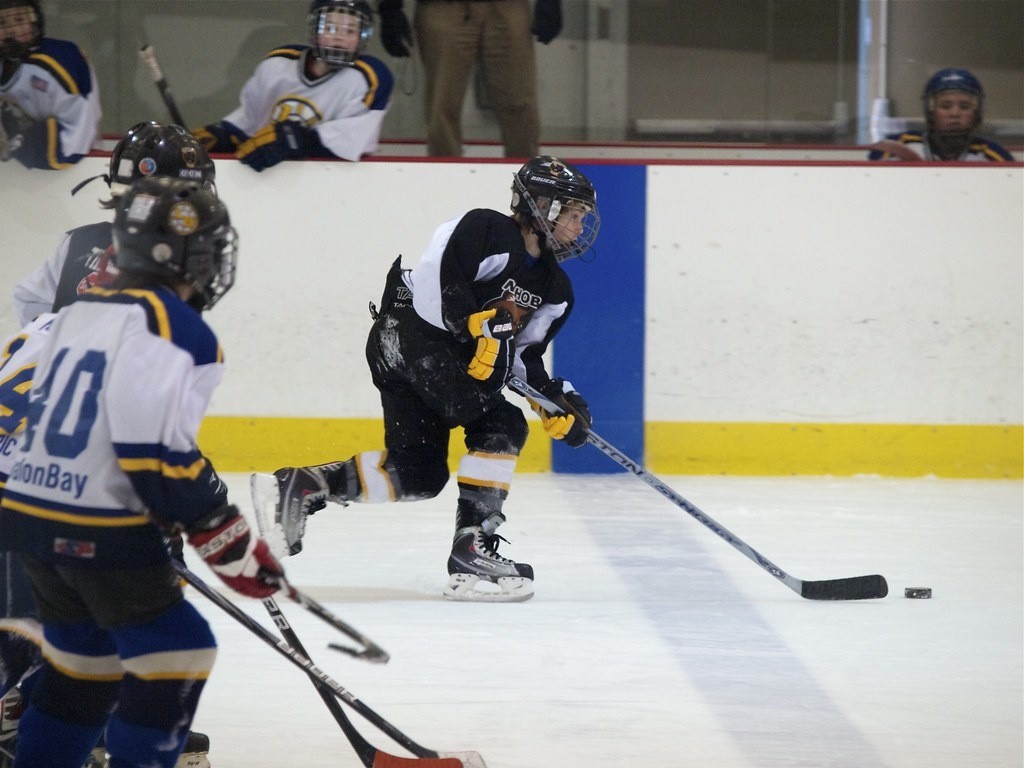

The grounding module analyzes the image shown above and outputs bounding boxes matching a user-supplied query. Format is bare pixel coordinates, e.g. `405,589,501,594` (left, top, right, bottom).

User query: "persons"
377,0,563,159
188,0,395,172
250,157,602,604
0,0,102,171
869,68,1015,162
0,118,285,768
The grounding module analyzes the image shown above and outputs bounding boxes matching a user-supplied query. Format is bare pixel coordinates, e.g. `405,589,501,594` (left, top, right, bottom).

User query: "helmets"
0,0,44,57
306,0,374,66
109,121,215,195
922,69,986,140
111,176,238,311
510,155,601,264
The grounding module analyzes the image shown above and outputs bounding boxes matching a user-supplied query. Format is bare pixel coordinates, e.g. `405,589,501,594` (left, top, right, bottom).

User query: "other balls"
904,587,932,599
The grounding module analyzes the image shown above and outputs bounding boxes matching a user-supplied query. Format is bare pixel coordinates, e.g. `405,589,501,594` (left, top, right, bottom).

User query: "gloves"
236,120,321,171
377,0,413,58
525,377,593,449
456,308,516,392
187,504,283,599
190,120,241,151
532,0,562,45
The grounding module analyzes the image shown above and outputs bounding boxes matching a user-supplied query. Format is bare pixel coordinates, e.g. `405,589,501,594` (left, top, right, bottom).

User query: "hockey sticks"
172,557,489,768
260,595,464,767
279,587,390,663
137,42,189,129
508,375,890,602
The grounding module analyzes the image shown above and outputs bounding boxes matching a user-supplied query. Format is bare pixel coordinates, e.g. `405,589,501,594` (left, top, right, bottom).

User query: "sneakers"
171,731,211,768
251,461,348,556
443,498,534,602
